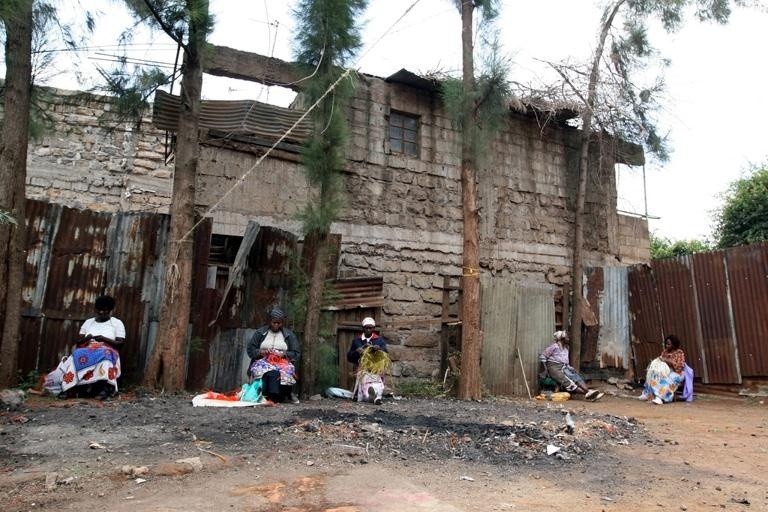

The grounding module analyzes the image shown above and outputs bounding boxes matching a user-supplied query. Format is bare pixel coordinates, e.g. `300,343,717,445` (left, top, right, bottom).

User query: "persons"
60,295,126,400
638,334,685,407
243,307,301,406
346,315,389,408
537,328,606,402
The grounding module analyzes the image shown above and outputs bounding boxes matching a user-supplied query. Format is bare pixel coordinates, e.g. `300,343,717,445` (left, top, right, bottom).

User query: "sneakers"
367,386,382,404
287,392,300,404
94,386,115,400
652,396,664,405
583,389,605,401
59,390,77,398
638,394,648,401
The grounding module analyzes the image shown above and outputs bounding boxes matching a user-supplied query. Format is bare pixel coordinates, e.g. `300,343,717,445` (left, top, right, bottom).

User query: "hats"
270,308,284,322
361,317,376,327
